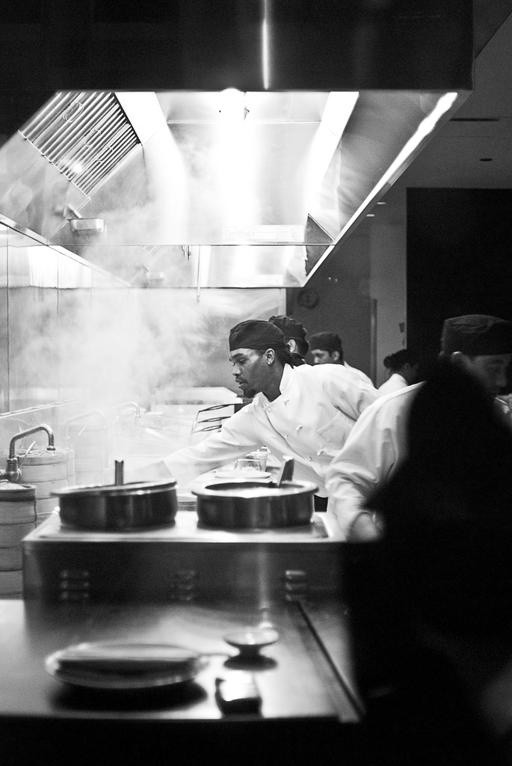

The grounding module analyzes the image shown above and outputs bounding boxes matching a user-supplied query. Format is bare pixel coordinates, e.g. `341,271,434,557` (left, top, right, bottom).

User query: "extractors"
1,84,174,256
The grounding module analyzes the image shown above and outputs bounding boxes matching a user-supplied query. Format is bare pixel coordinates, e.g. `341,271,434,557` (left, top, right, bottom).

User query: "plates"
224,628,280,651
43,641,207,693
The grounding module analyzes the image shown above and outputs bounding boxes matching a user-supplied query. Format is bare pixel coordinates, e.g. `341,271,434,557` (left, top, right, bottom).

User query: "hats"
229,315,342,351
440,315,512,356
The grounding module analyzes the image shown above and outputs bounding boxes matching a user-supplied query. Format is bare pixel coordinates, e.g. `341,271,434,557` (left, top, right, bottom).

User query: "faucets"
5,423,56,482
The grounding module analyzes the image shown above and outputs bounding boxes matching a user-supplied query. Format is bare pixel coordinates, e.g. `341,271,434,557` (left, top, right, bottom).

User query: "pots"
53,475,319,530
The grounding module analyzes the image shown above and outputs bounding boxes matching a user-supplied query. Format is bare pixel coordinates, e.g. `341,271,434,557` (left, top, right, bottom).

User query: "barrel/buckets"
16,446,74,523
68,422,109,488
0,483,37,599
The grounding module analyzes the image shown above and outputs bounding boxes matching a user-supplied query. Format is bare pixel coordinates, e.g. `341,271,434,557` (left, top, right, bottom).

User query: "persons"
126,318,380,513
271,315,419,395
325,314,512,766
